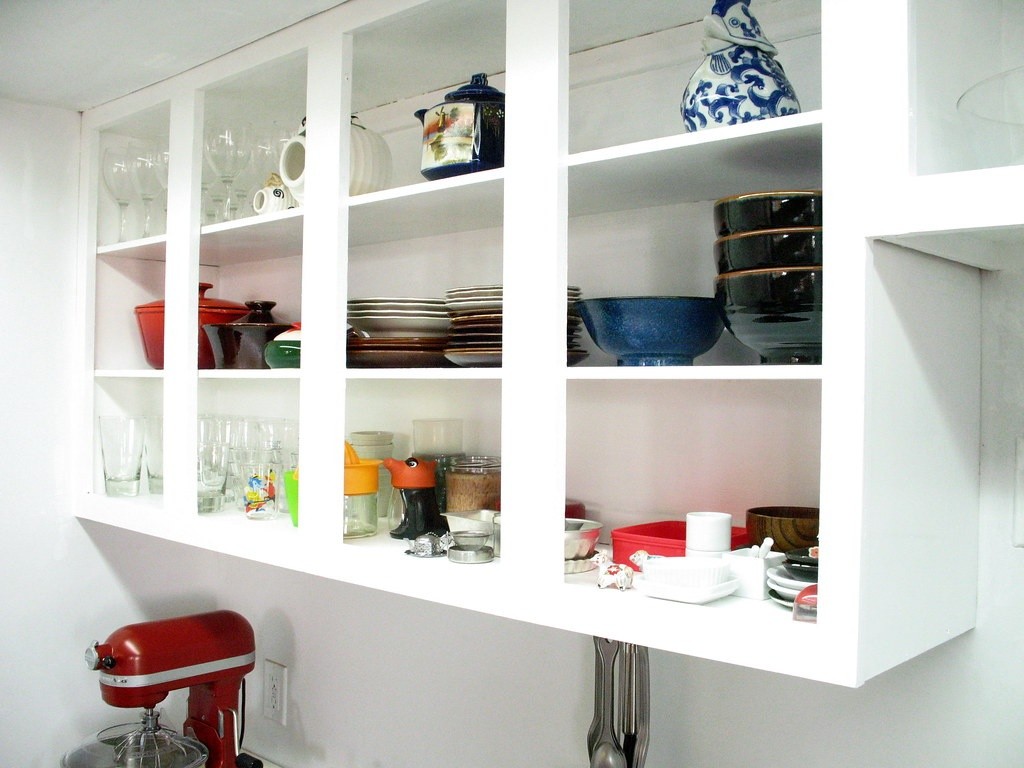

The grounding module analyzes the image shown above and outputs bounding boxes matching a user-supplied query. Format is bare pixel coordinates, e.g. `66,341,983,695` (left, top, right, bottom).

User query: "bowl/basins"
951,64,1024,166
745,506,819,552
201,300,292,370
712,189,823,364
135,281,251,371
565,518,603,560
571,296,724,366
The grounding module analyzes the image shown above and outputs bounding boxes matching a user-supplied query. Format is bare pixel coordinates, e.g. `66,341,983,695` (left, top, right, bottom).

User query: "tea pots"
413,73,504,180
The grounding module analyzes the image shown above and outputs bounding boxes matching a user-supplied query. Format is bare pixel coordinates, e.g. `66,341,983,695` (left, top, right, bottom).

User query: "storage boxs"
611,520,748,572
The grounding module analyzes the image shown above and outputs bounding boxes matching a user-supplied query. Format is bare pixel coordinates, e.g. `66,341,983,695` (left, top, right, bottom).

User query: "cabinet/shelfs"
79,1,1024,689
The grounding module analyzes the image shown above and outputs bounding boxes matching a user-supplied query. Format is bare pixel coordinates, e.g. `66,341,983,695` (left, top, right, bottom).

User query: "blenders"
344,441,384,538
57,610,263,768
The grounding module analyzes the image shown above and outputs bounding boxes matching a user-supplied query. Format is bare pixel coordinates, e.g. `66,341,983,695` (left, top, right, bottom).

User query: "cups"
143,413,163,495
723,547,785,600
686,511,731,559
196,413,298,528
351,416,464,535
99,416,144,496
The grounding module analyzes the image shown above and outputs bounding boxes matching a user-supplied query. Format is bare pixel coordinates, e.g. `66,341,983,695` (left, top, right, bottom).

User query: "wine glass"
102,115,295,243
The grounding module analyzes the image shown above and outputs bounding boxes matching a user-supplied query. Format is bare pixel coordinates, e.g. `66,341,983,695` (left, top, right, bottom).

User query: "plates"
766,549,818,608
443,285,590,367
346,297,451,368
638,558,740,604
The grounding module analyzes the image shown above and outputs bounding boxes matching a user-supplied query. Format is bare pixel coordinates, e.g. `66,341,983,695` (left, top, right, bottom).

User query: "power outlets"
262,660,288,727
